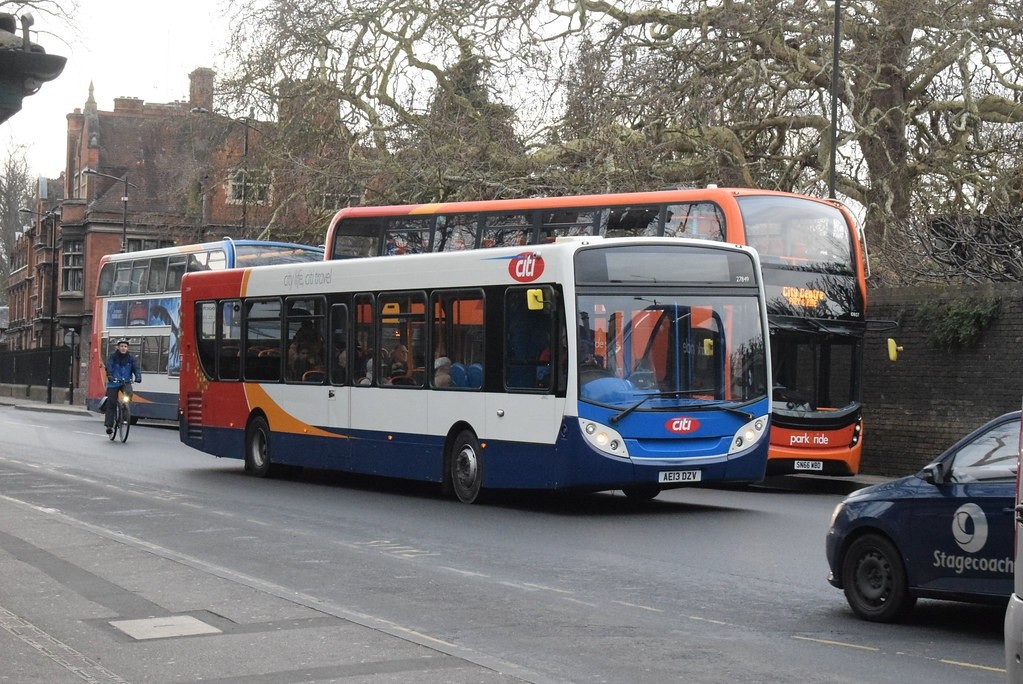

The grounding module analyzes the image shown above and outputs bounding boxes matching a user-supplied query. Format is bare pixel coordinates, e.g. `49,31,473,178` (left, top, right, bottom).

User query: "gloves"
135,378,141,383
108,376,115,383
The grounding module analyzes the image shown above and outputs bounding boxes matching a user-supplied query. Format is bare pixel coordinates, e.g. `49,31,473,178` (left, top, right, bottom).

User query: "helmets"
117,337,129,346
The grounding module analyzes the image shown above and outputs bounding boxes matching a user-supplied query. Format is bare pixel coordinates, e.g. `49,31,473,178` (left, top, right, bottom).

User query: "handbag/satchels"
98,396,108,412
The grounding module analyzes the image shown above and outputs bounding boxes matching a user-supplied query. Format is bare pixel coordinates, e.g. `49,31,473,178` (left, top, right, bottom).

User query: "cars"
826,408,1022,625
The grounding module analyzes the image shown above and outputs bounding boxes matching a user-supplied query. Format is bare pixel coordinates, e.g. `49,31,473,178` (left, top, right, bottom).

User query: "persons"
103,337,142,434
285,320,453,388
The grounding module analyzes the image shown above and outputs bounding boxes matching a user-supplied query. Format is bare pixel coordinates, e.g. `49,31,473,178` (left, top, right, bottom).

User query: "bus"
322,179,903,480
86,235,327,426
177,234,782,506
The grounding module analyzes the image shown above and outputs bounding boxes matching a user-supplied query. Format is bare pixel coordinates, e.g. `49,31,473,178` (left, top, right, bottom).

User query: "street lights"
19,207,56,402
190,106,249,240
82,166,140,252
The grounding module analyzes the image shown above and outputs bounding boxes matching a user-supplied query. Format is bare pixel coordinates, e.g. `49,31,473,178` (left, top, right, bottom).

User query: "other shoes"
106,428,113,434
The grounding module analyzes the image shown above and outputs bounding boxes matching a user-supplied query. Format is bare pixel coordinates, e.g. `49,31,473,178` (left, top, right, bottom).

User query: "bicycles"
108,378,139,443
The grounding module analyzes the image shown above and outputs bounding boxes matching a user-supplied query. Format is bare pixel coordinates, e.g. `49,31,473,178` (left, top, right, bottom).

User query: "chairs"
382,227,596,254
213,343,483,390
